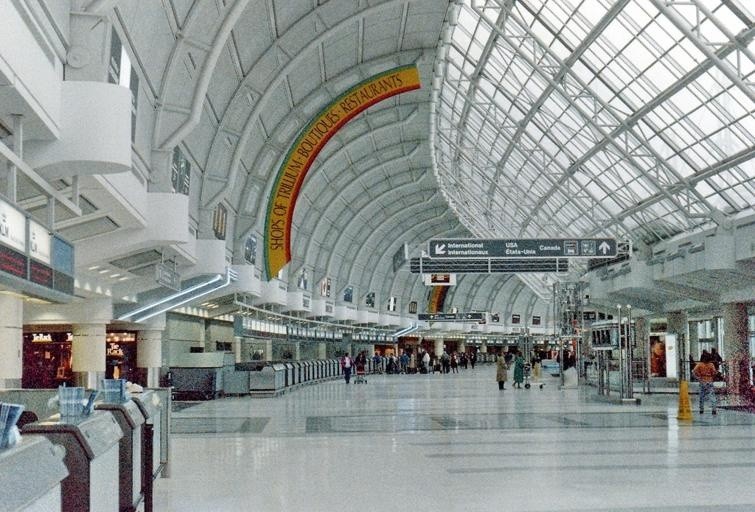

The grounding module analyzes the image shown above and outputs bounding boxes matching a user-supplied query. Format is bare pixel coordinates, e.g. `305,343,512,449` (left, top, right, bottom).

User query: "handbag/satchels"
713,376,727,390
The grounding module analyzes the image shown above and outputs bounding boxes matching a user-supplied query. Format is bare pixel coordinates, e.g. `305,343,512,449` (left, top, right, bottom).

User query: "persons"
691,355,717,414
341,348,476,384
496,346,541,390
556,350,576,370
690,348,722,381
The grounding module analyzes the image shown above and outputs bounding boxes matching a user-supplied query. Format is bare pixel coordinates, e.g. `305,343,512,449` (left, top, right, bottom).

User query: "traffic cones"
676,379,695,421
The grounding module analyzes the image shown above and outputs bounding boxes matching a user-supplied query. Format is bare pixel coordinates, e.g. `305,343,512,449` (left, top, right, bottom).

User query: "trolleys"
524,365,546,390
